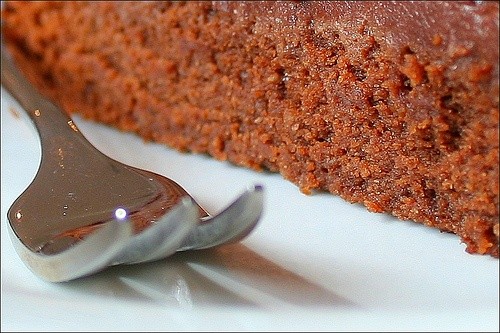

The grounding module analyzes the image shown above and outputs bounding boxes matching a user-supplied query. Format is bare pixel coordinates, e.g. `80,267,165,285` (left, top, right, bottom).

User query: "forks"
0,44,265,283
